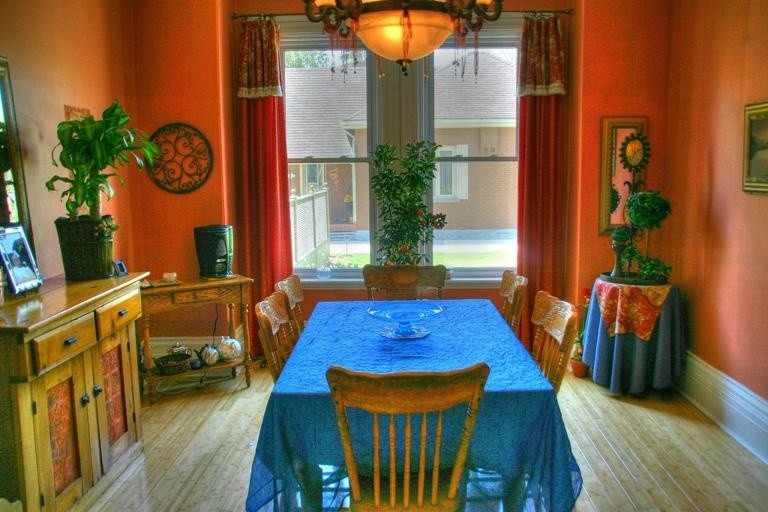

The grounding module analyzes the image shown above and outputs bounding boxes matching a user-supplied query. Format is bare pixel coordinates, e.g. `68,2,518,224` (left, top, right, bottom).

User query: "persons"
8,252,36,286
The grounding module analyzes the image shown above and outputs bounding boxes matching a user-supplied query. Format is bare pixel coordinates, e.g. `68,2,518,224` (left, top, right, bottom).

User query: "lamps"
301,0,498,88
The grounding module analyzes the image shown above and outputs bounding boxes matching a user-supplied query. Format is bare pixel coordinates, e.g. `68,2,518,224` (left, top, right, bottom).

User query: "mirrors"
598,111,649,239
0,52,40,284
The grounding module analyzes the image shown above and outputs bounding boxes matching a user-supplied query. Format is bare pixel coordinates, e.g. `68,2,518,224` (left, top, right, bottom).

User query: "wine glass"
365,300,443,340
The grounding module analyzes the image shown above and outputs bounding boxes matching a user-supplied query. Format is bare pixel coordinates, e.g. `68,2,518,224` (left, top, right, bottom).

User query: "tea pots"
191,343,222,366
165,342,193,357
217,338,242,362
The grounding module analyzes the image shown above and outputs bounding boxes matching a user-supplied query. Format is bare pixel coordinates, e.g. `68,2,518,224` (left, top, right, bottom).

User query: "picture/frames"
0,217,43,298
112,258,130,278
740,97,767,196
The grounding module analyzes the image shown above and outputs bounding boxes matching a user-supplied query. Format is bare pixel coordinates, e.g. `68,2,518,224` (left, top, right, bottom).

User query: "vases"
571,355,590,378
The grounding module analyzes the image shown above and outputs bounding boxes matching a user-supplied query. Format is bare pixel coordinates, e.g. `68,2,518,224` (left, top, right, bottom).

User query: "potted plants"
608,222,627,279
43,98,162,282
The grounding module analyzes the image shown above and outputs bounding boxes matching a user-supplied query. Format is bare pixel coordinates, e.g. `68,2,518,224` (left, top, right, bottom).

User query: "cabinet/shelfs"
0,270,156,512
134,269,256,407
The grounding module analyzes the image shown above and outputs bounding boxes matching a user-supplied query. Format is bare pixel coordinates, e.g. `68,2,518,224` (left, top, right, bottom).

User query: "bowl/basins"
162,273,177,282
154,352,193,375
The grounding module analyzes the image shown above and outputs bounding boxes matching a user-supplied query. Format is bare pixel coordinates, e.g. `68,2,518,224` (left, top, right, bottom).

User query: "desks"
579,271,686,399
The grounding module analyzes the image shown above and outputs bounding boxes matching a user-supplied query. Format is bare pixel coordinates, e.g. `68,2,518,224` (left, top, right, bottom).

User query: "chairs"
272,273,311,335
526,283,579,395
495,264,531,330
251,288,298,381
319,360,490,512
360,262,449,301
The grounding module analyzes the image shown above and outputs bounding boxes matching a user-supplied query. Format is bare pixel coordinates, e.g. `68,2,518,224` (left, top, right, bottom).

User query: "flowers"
571,287,592,362
